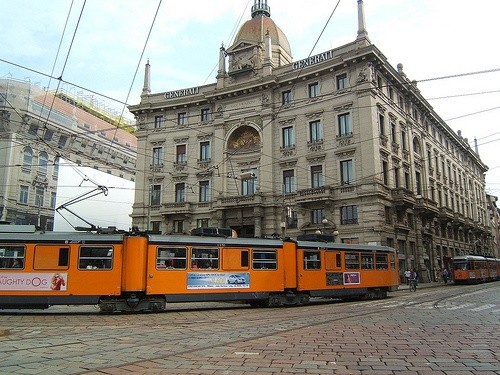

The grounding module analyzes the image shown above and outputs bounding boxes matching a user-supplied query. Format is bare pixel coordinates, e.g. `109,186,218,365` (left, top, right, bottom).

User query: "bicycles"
408,278,416,292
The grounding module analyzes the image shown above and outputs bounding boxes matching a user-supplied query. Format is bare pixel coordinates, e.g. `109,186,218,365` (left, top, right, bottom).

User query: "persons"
191,259,218,269
439,268,452,284
50,274,65,290
405,268,419,292
0,258,23,268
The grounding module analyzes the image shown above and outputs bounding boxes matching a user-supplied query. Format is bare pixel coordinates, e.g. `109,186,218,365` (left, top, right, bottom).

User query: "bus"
0,206,400,315
451,255,500,285
